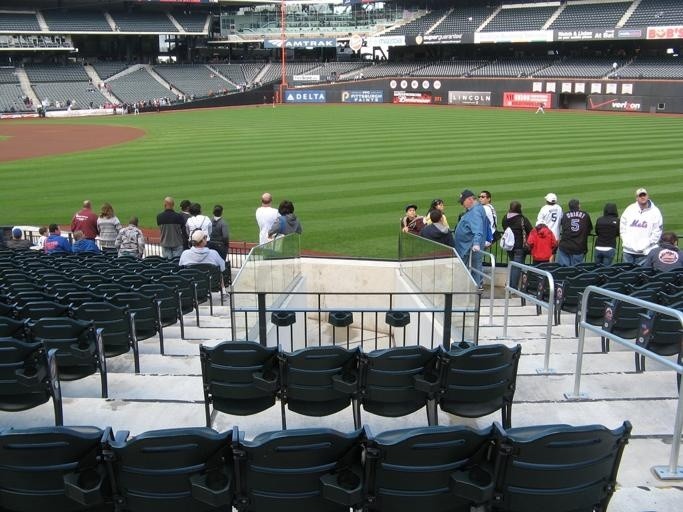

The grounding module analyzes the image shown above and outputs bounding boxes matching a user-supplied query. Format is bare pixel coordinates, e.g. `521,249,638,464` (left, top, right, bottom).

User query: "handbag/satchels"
491,228,502,245
519,216,534,254
257,216,287,254
498,226,517,253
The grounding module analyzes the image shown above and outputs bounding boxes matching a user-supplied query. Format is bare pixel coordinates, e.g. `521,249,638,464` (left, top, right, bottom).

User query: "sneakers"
477,283,484,290
219,290,231,302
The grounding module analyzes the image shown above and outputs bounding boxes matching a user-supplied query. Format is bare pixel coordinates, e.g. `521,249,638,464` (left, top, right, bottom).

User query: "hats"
456,189,474,204
404,203,417,212
179,199,190,209
11,228,22,237
187,228,205,242
635,187,647,196
543,192,558,204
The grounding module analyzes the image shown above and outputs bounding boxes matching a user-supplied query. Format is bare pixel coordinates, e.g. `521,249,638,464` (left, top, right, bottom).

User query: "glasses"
478,195,487,198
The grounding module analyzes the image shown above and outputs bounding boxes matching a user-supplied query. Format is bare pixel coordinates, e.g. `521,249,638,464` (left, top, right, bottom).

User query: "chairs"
0,1,257,111
0,421,632,510
520,260,682,396
378,0,683,81
0,245,231,430
257,61,377,84
200,339,521,431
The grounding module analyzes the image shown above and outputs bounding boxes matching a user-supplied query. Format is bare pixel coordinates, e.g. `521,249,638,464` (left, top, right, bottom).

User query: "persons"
267,201,301,239
400,188,683,292
613,62,617,71
7,196,230,302
256,192,281,247
536,104,544,114
1,76,263,119
